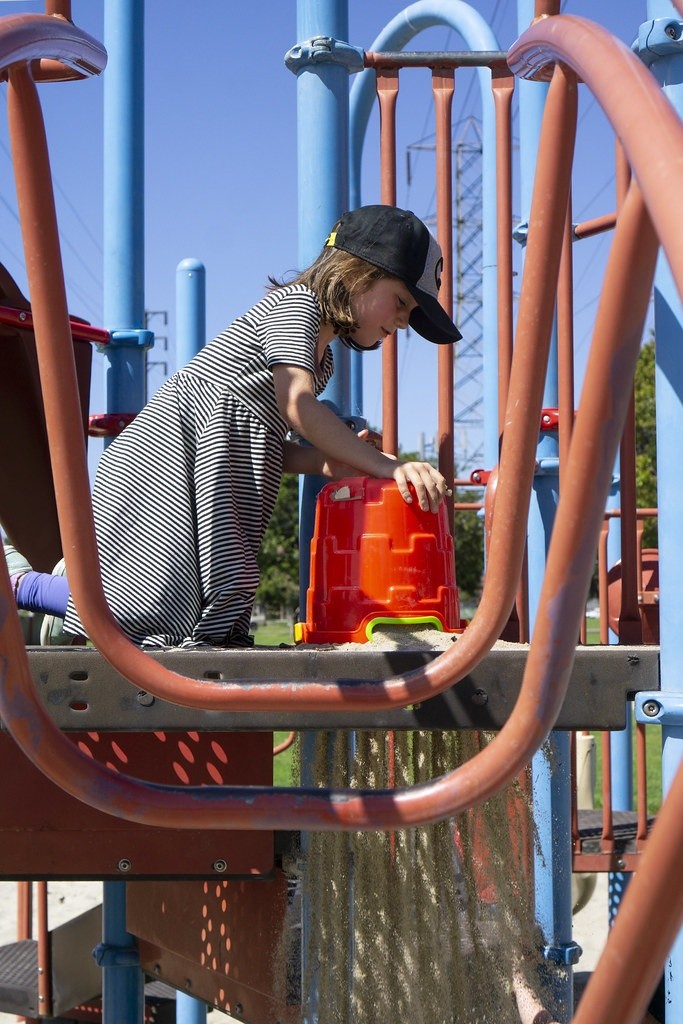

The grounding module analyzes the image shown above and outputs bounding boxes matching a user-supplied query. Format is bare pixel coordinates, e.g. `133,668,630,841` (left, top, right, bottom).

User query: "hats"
325,205,463,344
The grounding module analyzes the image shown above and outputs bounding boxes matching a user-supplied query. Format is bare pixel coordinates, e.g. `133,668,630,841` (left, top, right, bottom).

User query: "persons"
1,204,462,648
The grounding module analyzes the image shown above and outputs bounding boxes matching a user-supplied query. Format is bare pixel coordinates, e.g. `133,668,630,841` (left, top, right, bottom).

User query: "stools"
294,476,469,645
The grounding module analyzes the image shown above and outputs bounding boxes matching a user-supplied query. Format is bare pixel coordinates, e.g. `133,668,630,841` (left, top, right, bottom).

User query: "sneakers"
4,545,32,577
